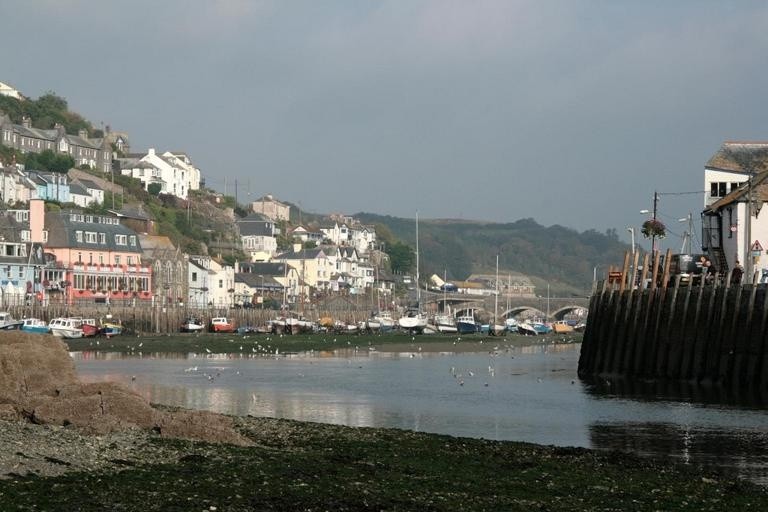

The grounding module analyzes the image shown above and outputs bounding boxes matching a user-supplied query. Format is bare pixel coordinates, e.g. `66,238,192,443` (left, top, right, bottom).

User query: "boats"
0,311,125,340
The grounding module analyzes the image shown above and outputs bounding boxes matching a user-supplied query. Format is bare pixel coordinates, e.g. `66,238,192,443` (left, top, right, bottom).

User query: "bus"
656,253,709,287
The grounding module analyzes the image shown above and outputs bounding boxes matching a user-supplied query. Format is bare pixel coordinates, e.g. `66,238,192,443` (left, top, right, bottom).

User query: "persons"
704,260,716,285
647,265,654,279
699,256,708,266
735,260,745,274
